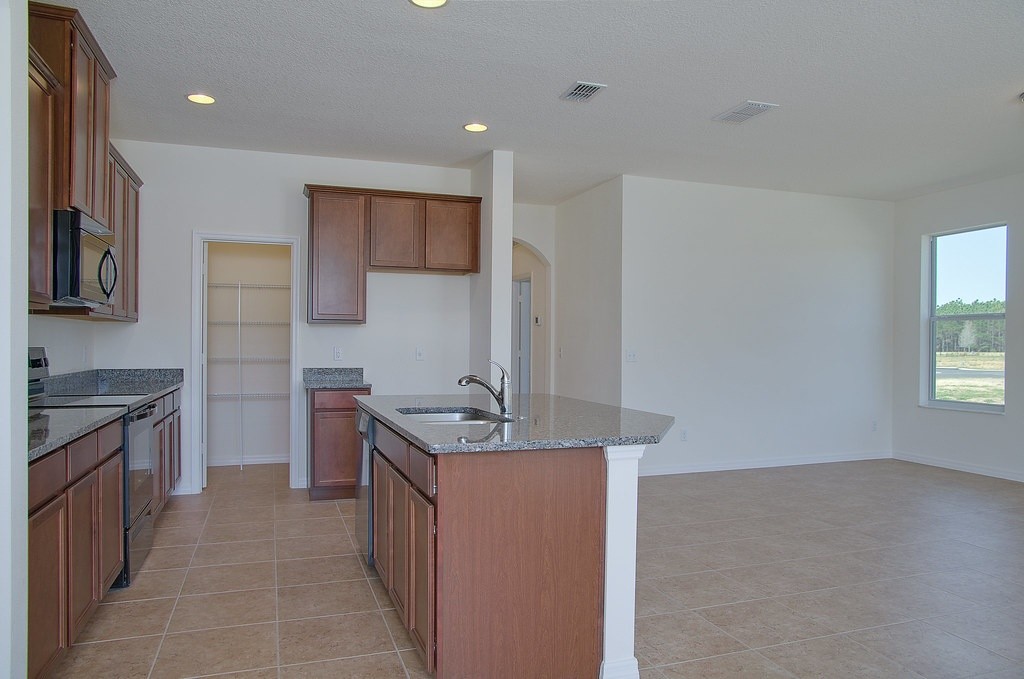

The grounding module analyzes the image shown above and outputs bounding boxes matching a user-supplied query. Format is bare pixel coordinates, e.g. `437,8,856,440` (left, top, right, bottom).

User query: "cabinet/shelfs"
28,418,124,678
148,388,183,524
28,1,144,323
368,417,609,679
302,184,483,325
305,388,371,501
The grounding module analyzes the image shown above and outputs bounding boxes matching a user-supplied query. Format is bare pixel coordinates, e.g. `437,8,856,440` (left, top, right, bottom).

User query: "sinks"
394,406,521,425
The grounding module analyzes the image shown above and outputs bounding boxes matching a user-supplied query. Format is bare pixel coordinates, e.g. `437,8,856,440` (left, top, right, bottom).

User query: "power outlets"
334,347,343,361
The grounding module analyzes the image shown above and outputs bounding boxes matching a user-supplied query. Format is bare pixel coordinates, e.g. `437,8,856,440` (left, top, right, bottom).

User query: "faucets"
457,422,508,444
457,359,514,415
